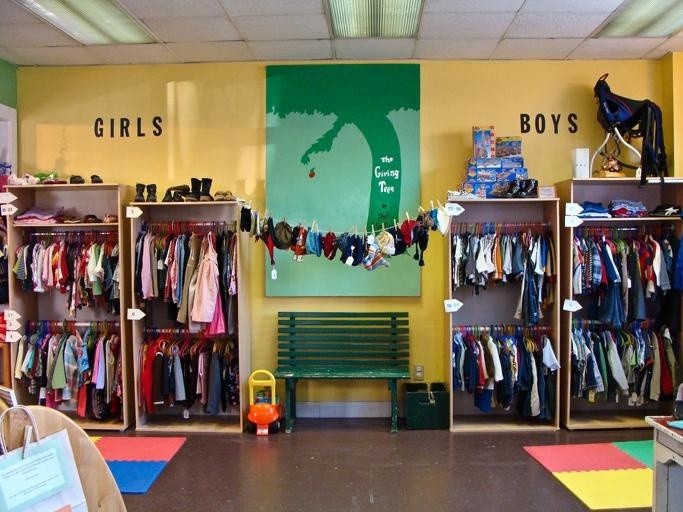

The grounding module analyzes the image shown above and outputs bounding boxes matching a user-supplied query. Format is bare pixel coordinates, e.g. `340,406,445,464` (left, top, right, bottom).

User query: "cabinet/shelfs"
2,183,135,433
448,195,561,432
127,199,252,434
554,177,683,431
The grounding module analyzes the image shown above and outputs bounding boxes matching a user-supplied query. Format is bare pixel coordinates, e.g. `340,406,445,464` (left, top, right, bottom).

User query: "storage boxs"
403,382,449,430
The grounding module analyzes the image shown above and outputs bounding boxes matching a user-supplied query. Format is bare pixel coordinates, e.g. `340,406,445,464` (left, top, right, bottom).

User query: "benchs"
273,311,410,432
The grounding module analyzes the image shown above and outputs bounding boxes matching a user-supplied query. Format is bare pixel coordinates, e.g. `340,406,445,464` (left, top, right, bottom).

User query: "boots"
147,184,157,201
134,183,146,202
191,178,201,201
502,178,538,198
199,179,213,201
162,185,189,202
172,190,189,202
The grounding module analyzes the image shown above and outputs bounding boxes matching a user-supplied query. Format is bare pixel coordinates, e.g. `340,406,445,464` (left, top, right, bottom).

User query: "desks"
644,415,683,512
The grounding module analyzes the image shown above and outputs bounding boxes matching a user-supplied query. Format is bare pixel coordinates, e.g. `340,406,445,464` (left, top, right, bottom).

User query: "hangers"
27,229,118,257
451,218,551,238
143,218,238,251
141,326,237,357
572,317,676,351
453,320,553,353
24,318,116,350
575,226,675,252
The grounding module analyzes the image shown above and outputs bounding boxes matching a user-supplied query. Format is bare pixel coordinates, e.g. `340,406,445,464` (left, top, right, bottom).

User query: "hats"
240,207,453,269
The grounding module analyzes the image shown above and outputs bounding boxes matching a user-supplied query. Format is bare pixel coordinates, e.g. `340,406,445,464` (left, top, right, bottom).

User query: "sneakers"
22,175,41,185
223,191,236,202
8,175,25,186
214,191,226,201
91,175,102,183
70,175,84,185
54,179,66,184
43,179,55,184
